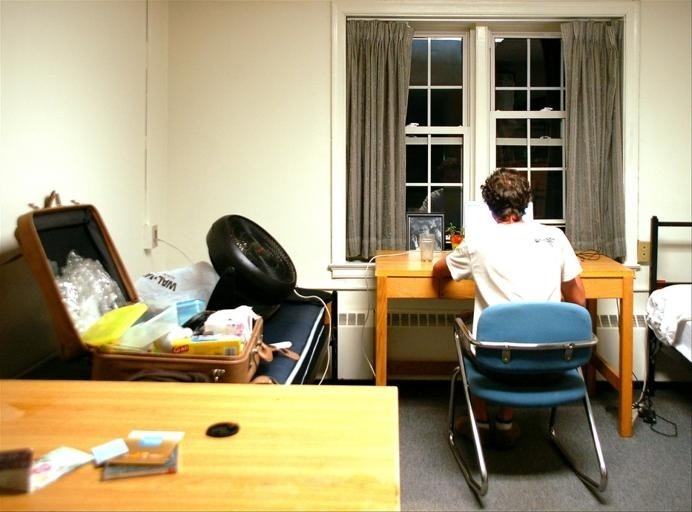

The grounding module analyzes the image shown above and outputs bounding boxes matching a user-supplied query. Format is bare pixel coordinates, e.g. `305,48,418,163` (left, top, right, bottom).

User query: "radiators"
337,308,648,380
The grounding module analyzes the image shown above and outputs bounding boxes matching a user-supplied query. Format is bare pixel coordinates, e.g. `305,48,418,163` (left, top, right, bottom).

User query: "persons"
417,157,462,214
431,167,589,449
411,220,439,250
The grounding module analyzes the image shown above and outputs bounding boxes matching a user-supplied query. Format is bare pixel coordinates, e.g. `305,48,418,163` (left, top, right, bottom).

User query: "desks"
0,378,401,512
372,247,635,439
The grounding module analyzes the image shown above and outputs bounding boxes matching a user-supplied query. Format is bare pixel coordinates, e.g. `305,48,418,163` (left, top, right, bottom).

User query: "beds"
10,288,340,384
645,214,692,396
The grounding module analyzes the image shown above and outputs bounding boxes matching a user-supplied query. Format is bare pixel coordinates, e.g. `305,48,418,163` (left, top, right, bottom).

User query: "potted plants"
445,222,465,250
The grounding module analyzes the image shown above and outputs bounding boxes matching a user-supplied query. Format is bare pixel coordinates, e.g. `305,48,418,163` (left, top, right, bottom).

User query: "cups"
419,233,435,262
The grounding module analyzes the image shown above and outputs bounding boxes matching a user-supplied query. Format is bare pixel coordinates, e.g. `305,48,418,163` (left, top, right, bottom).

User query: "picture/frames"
406,213,445,252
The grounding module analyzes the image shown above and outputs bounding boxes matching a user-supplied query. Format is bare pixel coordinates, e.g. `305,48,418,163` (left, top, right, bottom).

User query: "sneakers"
465,416,491,431
495,421,513,431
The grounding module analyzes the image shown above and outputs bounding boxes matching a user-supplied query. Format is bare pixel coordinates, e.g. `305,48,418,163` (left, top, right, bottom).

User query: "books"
100,442,178,479
110,430,185,464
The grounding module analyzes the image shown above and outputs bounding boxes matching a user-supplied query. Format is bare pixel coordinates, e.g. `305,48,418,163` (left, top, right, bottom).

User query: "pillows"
644,283,692,347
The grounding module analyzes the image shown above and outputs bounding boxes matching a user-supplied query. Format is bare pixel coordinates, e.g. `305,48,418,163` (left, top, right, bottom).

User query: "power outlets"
143,224,158,250
632,401,645,424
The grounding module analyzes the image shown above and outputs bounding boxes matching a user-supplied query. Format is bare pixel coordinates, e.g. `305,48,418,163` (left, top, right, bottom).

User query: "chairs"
444,301,609,497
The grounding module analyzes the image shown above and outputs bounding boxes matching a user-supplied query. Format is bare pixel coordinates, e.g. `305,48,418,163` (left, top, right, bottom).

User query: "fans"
204,215,298,312
